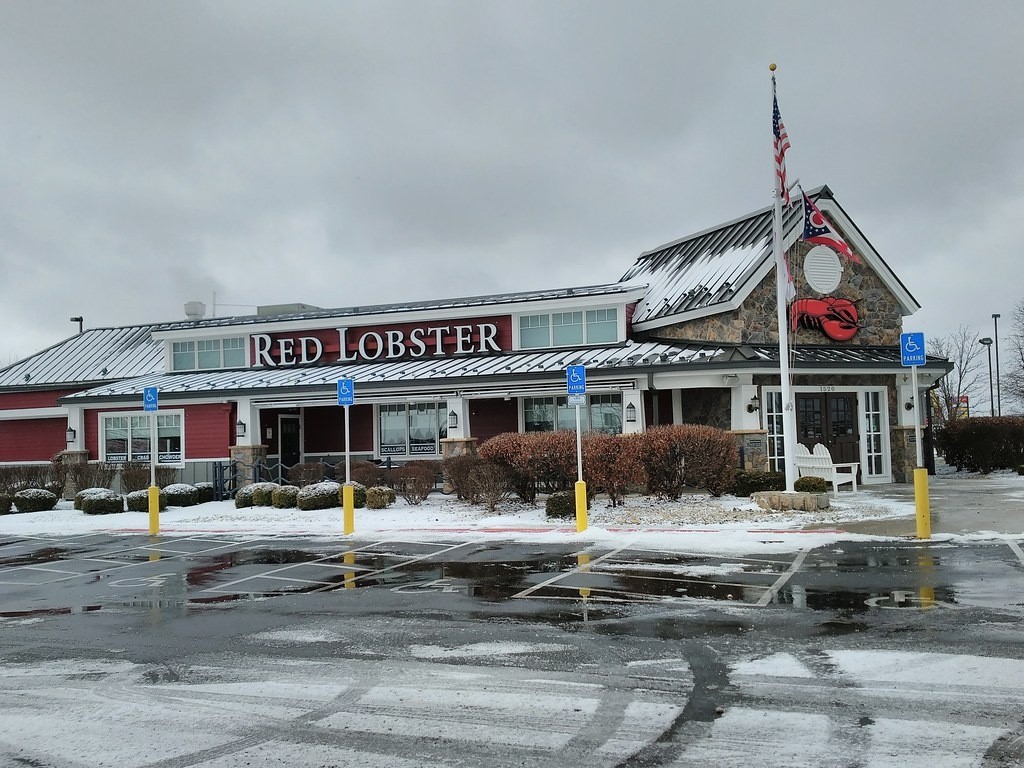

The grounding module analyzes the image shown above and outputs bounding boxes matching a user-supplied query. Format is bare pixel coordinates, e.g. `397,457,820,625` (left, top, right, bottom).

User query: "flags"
772,214,796,302
800,187,861,264
771,75,793,208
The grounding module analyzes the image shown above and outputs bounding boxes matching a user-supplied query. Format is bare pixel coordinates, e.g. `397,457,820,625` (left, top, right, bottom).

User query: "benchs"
794,443,860,499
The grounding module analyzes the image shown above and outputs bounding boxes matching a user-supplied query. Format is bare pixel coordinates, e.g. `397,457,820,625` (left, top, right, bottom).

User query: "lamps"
236,420,246,437
449,411,458,429
66,426,76,442
905,395,913,410
626,402,636,423
746,395,759,414
